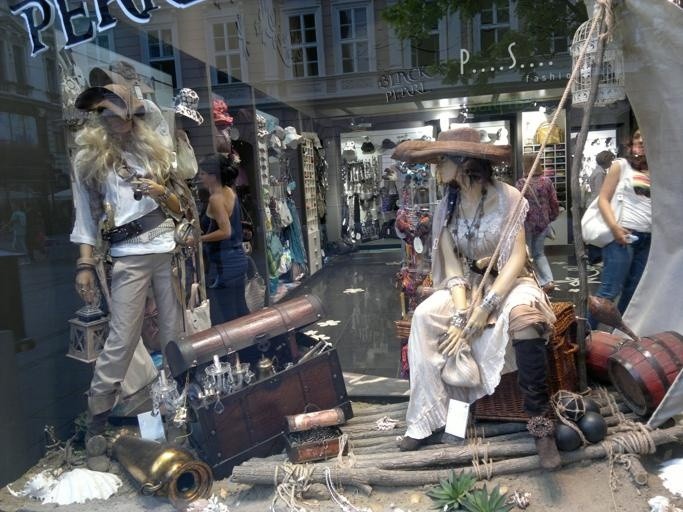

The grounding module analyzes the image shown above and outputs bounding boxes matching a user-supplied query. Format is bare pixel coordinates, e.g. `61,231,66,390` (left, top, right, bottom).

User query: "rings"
443,332,447,338
80,288,87,295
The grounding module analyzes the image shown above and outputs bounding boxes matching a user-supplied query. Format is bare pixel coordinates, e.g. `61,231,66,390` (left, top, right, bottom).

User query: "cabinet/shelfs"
522,142,569,250
301,136,324,277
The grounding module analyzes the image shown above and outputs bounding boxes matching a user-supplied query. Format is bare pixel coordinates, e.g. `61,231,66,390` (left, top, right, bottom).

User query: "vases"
100,419,215,509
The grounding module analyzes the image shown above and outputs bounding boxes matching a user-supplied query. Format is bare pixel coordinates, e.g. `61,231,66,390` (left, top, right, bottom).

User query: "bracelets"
480,290,503,315
450,315,464,328
159,185,170,202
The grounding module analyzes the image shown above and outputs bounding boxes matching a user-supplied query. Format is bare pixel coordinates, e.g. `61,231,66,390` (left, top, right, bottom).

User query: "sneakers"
399,424,448,451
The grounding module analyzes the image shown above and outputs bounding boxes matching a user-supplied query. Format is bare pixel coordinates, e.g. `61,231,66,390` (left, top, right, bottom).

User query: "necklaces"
453,185,487,242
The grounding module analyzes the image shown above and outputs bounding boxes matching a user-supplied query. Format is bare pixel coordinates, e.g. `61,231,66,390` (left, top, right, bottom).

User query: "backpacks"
536,121,564,147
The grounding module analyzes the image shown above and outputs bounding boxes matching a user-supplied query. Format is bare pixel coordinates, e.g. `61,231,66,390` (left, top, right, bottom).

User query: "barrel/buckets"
611,330,683,418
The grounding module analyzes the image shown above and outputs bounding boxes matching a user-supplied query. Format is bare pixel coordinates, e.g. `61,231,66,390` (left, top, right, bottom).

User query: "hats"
170,87,204,126
212,98,234,125
254,114,304,154
343,138,395,152
74,82,146,123
390,127,513,164
89,59,154,96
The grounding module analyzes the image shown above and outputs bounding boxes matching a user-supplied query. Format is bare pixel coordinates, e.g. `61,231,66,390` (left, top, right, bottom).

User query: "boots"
512,336,563,474
84,408,111,473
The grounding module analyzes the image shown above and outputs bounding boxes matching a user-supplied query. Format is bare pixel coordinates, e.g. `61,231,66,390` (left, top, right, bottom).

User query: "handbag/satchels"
580,157,624,248
438,332,481,389
268,182,294,229
382,180,400,212
243,254,266,314
184,282,212,337
544,222,556,241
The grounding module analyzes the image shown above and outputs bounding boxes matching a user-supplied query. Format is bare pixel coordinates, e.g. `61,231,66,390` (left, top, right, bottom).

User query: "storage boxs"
275,405,351,467
157,288,357,476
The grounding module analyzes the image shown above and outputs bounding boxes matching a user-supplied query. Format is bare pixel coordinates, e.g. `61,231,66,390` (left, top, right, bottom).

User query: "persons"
587,150,615,263
514,151,559,293
390,127,562,472
73,60,184,454
583,117,652,331
197,153,250,326
